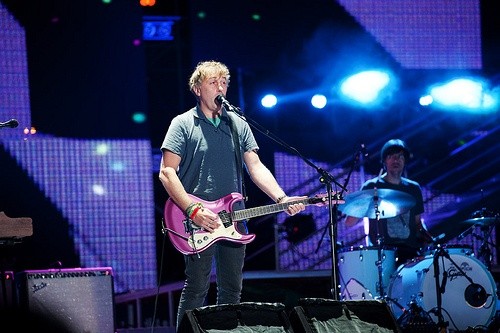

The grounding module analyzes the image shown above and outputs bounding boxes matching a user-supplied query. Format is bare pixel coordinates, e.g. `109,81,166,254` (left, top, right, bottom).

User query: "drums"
386,252,497,331
338,246,398,302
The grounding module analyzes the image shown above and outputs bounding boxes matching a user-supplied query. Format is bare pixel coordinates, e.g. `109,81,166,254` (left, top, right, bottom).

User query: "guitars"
164,189,346,255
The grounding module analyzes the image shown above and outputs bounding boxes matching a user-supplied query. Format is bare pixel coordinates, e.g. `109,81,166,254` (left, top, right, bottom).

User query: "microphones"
0,118,19,128
217,95,234,112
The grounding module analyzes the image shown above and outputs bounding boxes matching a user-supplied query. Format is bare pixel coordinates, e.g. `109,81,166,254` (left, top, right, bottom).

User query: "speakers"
290,297,400,333
0,271,21,333
15,267,113,333
178,303,293,333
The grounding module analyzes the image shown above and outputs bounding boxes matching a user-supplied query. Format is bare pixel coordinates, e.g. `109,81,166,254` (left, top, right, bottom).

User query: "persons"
342,140,424,269
159,60,307,330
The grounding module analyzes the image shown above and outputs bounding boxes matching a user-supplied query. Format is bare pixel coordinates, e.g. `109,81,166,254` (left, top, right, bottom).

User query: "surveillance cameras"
441,272,447,293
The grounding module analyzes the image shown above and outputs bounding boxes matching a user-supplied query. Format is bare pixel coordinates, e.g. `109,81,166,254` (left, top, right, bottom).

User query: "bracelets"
185,202,203,218
276,195,287,202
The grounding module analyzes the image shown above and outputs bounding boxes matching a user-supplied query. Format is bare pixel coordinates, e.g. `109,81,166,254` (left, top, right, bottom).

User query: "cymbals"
463,217,500,225
338,188,417,219
